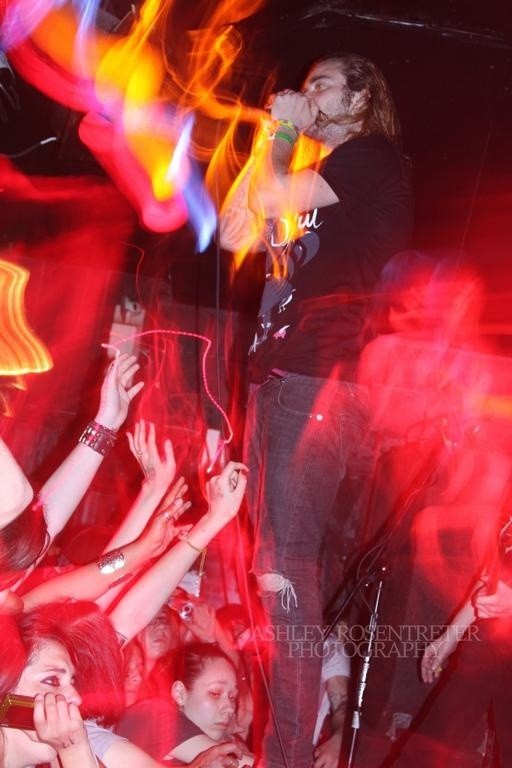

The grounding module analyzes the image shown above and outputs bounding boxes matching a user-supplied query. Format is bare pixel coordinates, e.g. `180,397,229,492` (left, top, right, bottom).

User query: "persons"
0,347,511,767
217,49,411,767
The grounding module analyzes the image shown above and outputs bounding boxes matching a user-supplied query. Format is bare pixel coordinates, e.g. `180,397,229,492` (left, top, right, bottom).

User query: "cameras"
1,694,37,731
228,752,256,768
167,596,196,622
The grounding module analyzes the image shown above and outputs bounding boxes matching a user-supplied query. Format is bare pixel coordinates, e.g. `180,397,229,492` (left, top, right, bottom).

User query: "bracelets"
270,117,300,137
267,129,293,145
